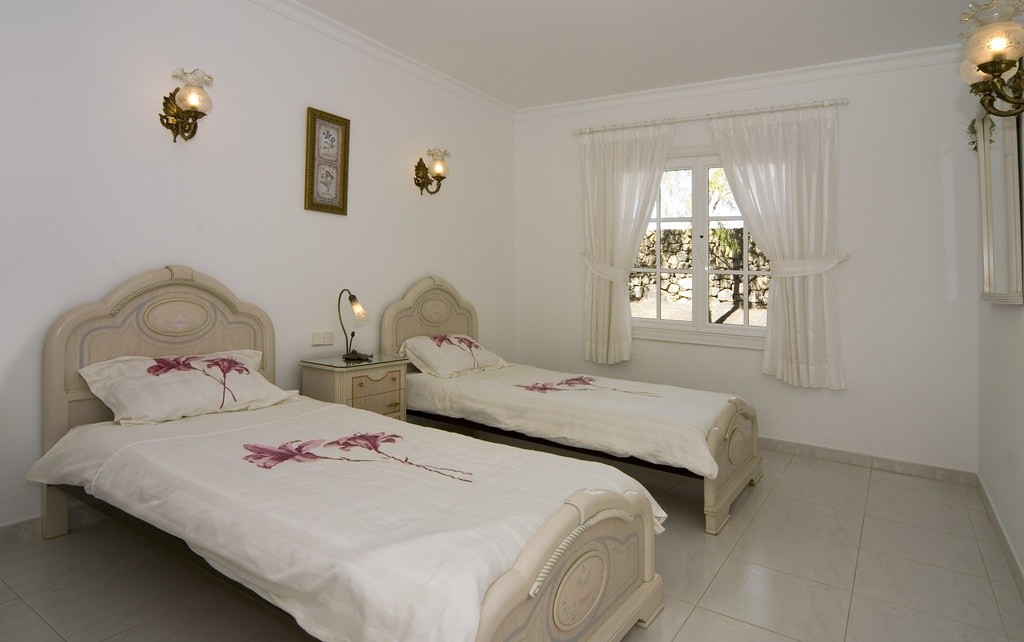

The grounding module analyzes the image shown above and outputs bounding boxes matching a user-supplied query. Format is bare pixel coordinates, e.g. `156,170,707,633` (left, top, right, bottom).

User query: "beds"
379,274,766,536
25,265,670,641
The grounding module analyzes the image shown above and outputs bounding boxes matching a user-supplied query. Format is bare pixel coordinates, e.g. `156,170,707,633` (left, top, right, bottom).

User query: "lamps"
337,289,373,362
158,67,215,142
958,1,1024,106
413,147,450,195
959,25,1024,118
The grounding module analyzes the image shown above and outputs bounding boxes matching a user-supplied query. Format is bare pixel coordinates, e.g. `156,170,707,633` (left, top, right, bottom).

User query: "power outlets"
312,332,324,345
323,332,335,345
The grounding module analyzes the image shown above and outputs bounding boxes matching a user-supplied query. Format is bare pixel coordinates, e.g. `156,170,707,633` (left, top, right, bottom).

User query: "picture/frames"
303,106,351,216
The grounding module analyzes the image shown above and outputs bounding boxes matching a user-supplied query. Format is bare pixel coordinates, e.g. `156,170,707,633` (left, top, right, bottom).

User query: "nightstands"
299,351,412,422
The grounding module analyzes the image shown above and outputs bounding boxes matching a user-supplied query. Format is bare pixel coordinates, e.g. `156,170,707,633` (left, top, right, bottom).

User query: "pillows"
399,333,512,378
76,349,300,426
